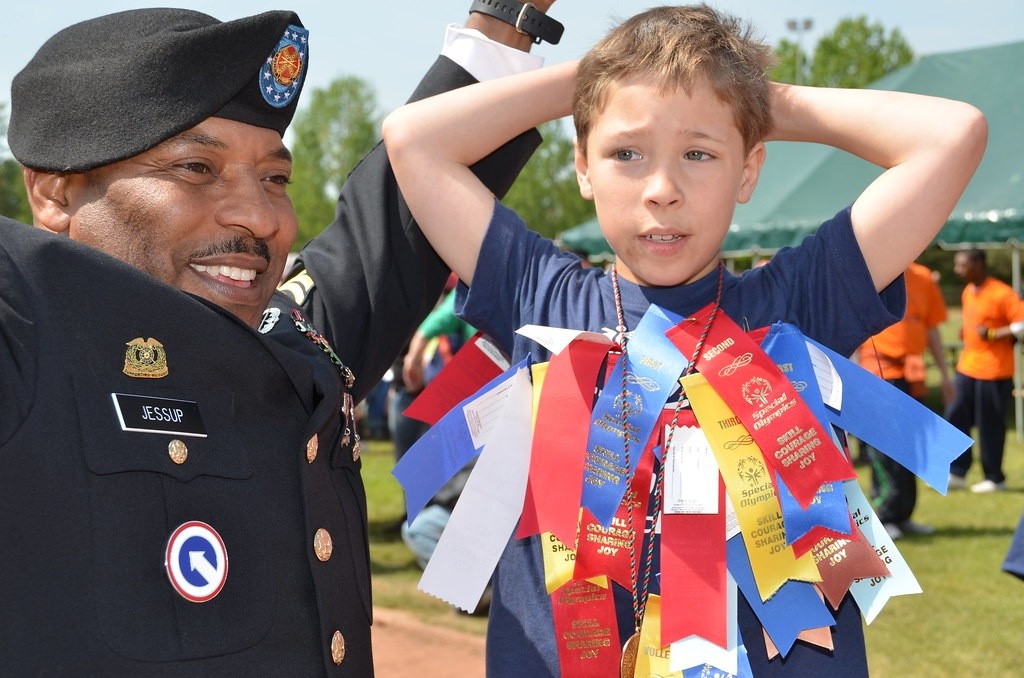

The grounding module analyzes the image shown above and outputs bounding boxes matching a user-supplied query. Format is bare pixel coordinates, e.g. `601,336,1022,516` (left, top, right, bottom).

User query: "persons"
850,262,957,541
379,1,989,678
0,0,560,678
388,277,499,617
925,249,1024,494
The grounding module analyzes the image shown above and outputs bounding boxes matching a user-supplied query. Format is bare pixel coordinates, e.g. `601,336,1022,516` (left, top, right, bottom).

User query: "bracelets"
987,328,996,340
469,0,565,46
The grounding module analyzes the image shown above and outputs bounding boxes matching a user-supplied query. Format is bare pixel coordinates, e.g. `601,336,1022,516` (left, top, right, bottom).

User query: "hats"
6,8,308,171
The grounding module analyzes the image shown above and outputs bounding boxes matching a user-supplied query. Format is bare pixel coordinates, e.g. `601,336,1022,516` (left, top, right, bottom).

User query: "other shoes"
970,480,1006,493
926,473,965,489
883,519,934,541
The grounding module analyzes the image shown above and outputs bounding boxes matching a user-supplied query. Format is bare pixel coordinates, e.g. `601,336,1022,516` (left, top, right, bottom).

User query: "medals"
619,626,641,678
